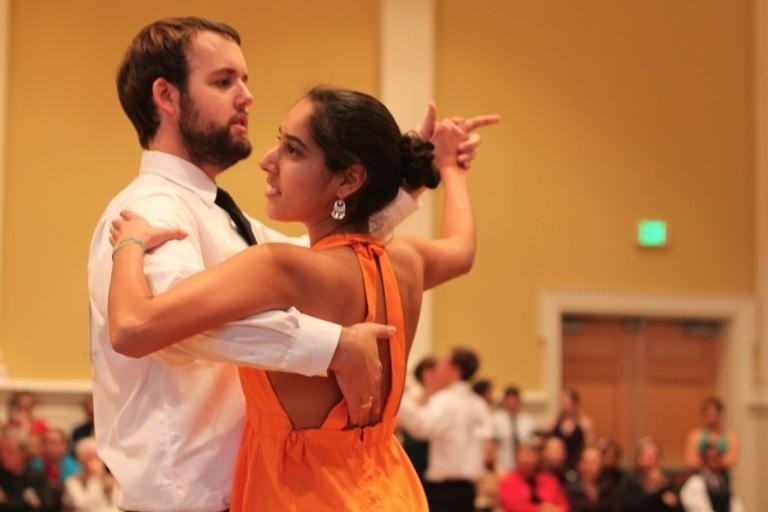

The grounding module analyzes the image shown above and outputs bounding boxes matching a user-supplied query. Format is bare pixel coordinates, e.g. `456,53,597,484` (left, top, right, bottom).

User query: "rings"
361,396,373,408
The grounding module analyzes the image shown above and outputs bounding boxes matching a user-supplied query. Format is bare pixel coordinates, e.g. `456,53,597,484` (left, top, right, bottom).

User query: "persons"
1,390,119,512
102,78,480,512
82,14,505,512
393,344,743,512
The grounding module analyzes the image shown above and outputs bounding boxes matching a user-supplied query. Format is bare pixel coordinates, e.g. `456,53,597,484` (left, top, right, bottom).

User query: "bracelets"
111,237,147,262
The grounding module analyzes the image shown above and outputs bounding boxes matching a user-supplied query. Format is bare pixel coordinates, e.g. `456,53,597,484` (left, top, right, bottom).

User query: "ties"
526,476,539,504
215,187,257,247
511,415,519,457
